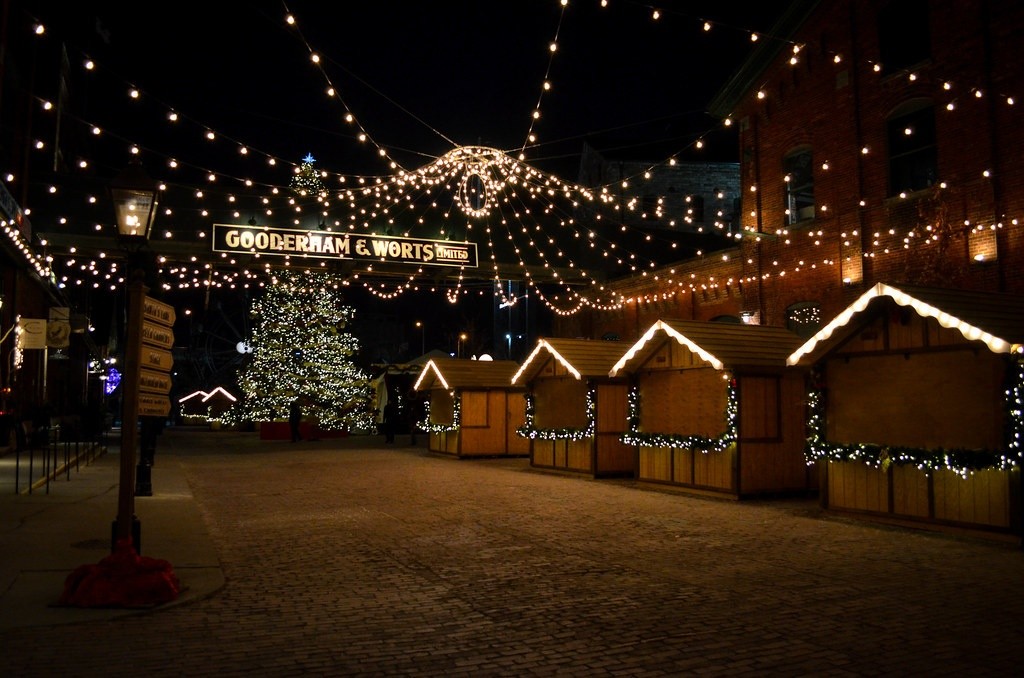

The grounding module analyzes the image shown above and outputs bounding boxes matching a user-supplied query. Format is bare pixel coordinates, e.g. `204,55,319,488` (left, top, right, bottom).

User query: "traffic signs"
142,293,177,327
137,391,172,417
141,318,176,350
137,367,175,396
138,343,175,373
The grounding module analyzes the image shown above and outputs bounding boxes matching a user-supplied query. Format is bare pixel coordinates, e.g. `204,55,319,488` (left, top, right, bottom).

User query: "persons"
407,400,425,445
289,402,302,443
383,400,396,444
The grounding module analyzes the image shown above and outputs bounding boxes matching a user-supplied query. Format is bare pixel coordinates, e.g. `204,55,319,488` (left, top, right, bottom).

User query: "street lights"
19,314,95,463
54,170,186,612
